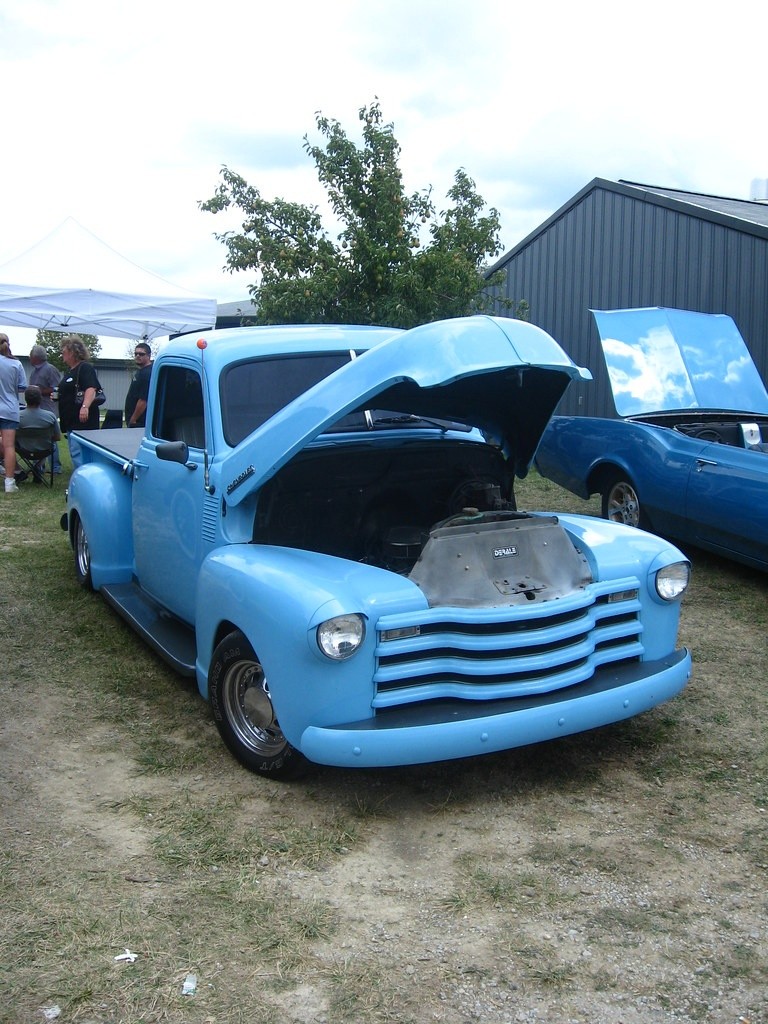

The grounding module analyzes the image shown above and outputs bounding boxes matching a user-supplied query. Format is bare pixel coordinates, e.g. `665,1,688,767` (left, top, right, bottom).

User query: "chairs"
102,409,124,430
13,425,57,490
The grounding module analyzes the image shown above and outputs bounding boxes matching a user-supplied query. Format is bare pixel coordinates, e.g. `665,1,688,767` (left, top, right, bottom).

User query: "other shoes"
0,464,6,475
5,478,19,492
14,471,29,485
33,464,43,484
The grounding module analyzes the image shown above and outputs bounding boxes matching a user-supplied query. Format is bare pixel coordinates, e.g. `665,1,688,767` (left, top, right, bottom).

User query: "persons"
124,343,152,428
0,332,27,492
58,336,100,468
0,385,61,484
30,345,63,473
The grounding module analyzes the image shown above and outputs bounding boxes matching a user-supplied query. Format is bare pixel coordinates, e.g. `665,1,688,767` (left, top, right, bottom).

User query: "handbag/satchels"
74,362,106,407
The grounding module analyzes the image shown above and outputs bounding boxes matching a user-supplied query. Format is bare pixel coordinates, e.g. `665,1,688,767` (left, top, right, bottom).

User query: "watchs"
81,404,88,408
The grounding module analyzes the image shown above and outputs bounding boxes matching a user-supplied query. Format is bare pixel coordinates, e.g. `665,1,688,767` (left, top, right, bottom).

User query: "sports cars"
533,307,768,572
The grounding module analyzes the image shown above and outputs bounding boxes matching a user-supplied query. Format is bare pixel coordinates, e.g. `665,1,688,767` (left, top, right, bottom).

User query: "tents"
0,285,217,343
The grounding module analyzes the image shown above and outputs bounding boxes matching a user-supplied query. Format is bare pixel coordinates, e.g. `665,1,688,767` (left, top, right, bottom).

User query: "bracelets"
129,421,136,425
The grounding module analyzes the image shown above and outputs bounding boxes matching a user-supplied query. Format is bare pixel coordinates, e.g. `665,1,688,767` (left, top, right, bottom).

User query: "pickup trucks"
60,315,693,783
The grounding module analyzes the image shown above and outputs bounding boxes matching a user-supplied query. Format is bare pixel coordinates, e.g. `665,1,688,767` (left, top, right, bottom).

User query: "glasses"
135,352,148,356
